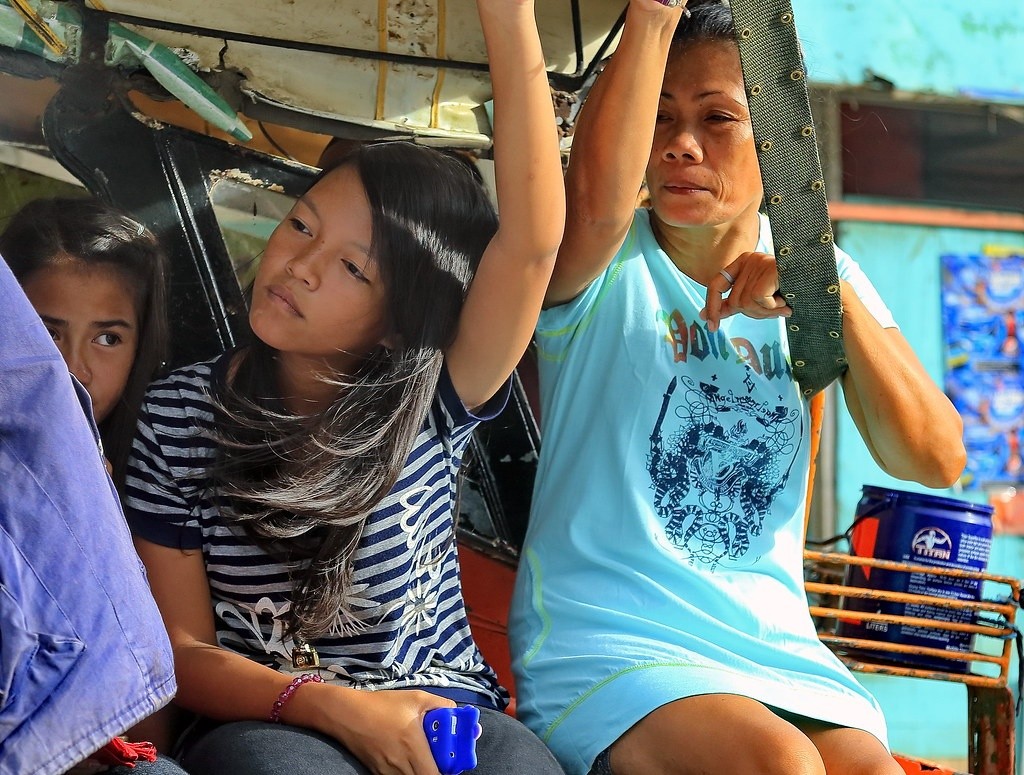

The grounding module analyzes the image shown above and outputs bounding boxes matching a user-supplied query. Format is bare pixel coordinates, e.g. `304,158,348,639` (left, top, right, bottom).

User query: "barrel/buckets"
835,484,995,674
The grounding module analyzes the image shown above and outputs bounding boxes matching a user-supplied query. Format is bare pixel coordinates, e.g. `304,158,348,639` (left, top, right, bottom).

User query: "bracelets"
267,670,327,718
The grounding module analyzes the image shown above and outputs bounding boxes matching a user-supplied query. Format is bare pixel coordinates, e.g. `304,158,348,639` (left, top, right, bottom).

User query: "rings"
718,269,734,283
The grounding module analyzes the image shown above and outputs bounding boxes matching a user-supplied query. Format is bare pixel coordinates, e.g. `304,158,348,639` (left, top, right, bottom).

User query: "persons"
509,0,969,775
127,1,569,773
0,249,178,775
1,195,175,507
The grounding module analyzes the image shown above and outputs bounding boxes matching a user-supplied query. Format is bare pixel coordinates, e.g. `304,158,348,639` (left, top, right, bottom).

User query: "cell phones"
422,704,482,775
